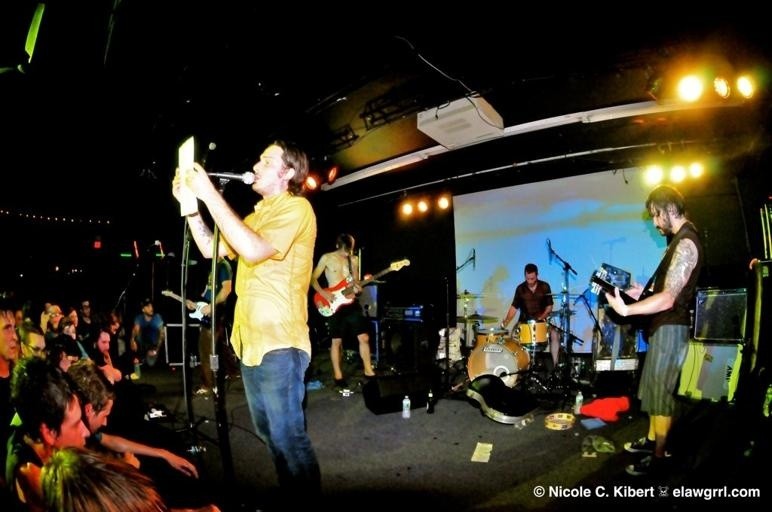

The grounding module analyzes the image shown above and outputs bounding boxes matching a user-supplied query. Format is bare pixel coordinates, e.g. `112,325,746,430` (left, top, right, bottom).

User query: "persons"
497,263,559,369
185,253,234,395
605,185,704,476
1,292,230,511
310,234,376,391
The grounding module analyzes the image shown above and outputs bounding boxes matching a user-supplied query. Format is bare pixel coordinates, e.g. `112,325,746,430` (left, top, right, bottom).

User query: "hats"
141,298,152,307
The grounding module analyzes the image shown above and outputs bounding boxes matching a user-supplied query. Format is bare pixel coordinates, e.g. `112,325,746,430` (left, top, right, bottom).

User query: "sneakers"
363,374,377,379
334,377,348,388
625,438,656,454
192,384,218,395
626,456,663,475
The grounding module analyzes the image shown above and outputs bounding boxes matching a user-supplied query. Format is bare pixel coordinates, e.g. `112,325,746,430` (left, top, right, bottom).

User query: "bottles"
426,388,434,414
401,395,411,420
574,391,584,416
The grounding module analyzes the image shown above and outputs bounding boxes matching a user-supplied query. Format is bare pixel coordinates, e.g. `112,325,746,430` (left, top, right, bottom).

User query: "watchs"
172,135,326,512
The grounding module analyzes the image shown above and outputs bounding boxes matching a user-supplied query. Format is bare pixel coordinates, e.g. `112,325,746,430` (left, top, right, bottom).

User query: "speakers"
362,375,420,414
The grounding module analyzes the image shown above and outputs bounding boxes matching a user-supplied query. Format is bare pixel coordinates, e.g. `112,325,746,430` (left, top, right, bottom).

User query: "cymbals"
546,292,582,298
458,293,484,300
457,314,498,322
548,310,575,314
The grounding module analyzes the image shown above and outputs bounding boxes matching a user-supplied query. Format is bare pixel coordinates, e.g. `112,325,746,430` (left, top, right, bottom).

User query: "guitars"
590,266,653,343
314,259,410,317
161,289,221,329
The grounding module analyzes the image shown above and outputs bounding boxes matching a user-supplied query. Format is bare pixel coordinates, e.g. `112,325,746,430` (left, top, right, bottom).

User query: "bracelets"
622,306,628,316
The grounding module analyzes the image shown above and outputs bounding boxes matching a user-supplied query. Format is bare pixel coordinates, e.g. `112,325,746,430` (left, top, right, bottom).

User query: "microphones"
473,249,476,269
208,172,257,185
548,241,552,265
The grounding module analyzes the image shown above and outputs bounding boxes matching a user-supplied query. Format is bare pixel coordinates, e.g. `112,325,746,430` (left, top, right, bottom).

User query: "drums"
477,328,509,348
466,339,531,386
518,318,548,348
